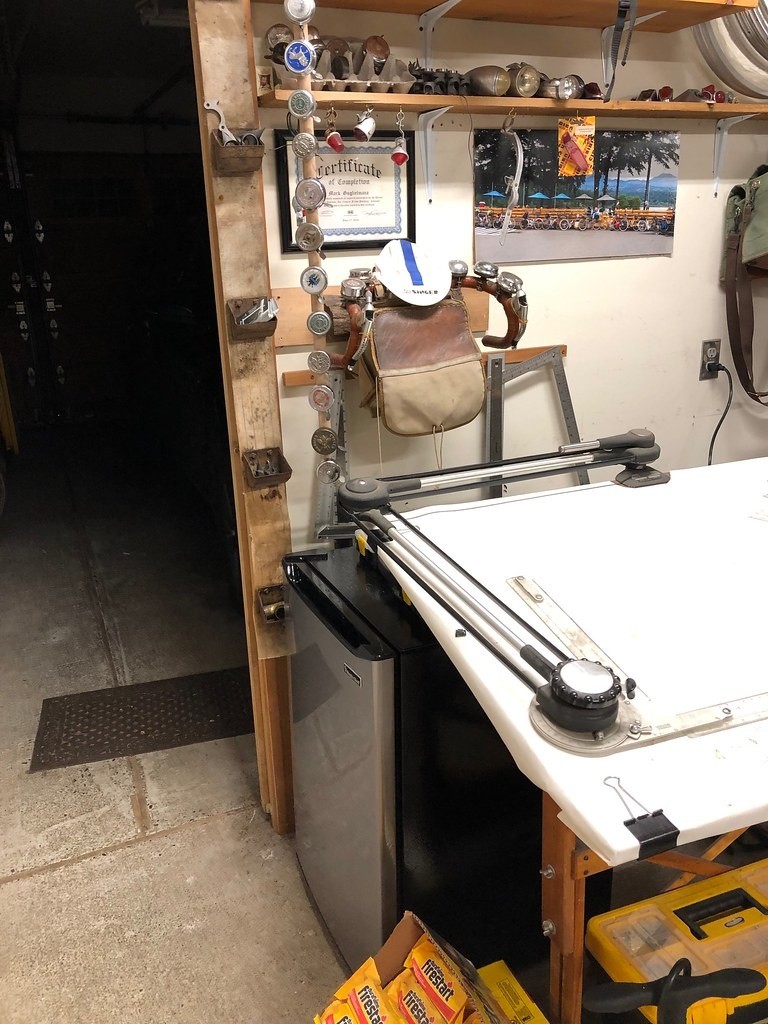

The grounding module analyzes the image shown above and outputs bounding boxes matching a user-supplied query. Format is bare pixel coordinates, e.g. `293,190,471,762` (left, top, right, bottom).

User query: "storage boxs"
310,911,513,1024
584,854,768,1024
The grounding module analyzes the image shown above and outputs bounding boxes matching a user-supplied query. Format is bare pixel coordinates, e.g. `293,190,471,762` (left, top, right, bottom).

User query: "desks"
370,455,768,1024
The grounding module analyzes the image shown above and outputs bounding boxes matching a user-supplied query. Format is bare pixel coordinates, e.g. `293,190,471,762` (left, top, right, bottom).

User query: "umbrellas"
553,193,571,200
483,191,506,198
597,194,616,201
576,194,593,200
528,192,550,200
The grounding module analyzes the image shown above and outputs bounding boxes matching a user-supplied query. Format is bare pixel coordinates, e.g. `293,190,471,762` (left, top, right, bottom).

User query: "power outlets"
698,338,723,381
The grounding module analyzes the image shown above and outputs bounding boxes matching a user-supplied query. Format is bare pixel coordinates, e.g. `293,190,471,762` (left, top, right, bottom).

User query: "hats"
373,239,453,305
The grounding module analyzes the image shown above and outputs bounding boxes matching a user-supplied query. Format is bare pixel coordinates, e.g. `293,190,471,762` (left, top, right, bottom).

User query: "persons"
486,208,493,221
644,200,649,210
588,207,612,218
522,210,529,219
475,207,481,217
661,219,667,229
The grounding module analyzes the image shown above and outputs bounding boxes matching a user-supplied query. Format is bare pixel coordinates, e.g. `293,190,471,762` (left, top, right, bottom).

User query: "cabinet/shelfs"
253,0,768,196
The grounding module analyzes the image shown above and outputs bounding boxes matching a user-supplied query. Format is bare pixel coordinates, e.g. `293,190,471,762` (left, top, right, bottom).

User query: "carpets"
26,660,255,775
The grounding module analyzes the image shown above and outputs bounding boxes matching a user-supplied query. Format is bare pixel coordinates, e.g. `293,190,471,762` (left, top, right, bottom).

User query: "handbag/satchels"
719,164,768,283
359,299,487,437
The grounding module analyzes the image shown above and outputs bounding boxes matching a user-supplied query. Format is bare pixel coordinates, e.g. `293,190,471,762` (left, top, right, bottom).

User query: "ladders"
0,101,71,428
